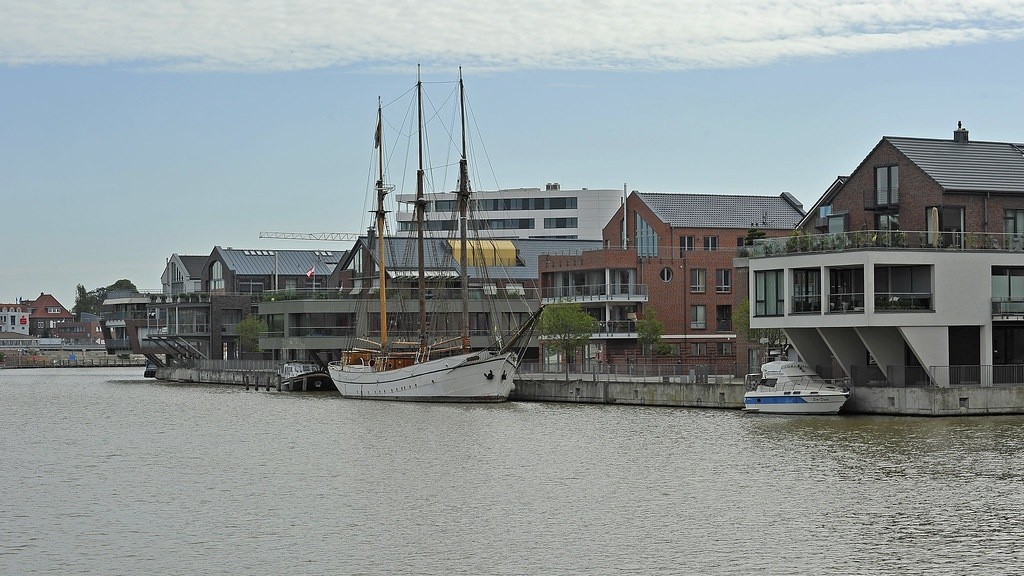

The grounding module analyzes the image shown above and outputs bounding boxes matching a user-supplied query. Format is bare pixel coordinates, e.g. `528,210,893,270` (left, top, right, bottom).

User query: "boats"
277,360,330,391
144,359,158,378
742,345,855,415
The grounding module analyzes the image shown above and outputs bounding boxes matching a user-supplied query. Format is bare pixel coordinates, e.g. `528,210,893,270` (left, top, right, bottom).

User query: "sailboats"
327,64,546,403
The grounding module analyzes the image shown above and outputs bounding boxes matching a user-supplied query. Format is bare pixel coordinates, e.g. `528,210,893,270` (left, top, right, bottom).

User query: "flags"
306,266,314,276
